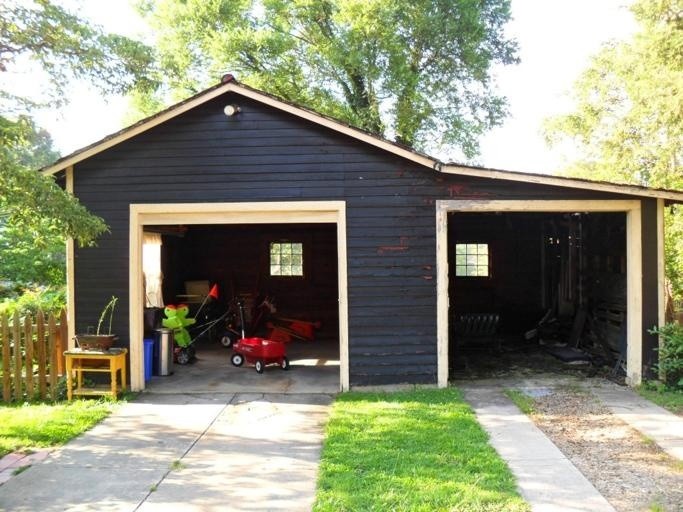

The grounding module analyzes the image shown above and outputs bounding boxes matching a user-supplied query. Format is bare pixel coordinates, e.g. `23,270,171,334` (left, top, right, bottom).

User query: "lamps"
223,103,242,116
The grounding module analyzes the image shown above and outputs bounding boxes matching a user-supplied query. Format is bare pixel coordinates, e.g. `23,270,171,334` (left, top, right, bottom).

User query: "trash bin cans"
151,327,174,376
144,339,154,383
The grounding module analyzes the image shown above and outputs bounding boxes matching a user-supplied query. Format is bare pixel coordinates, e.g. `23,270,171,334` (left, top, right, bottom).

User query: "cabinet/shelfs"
63,347,128,401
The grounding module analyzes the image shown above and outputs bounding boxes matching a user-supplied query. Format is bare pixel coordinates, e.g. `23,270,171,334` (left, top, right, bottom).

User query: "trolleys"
167,292,292,376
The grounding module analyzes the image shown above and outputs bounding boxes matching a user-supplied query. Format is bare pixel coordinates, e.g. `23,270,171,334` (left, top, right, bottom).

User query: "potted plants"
75,294,119,351
